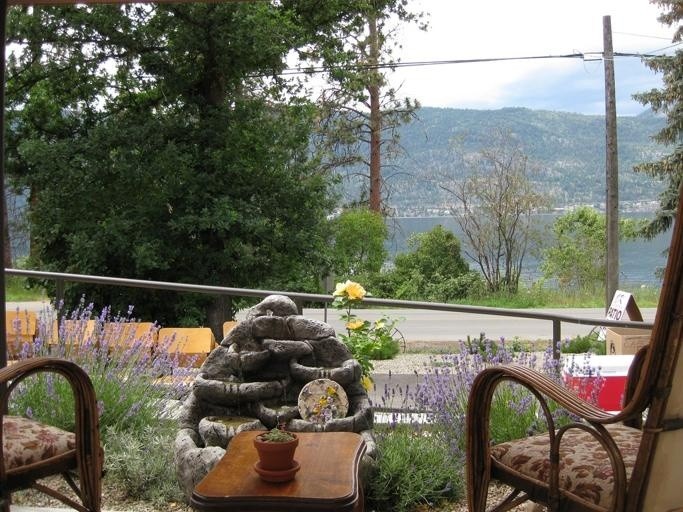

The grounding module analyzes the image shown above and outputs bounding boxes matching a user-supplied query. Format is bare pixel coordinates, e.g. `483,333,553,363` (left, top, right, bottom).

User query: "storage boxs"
606,327,653,354
560,354,635,411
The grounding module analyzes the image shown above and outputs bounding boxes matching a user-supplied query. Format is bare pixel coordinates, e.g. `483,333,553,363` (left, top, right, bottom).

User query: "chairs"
0,356,104,512
465,177,683,512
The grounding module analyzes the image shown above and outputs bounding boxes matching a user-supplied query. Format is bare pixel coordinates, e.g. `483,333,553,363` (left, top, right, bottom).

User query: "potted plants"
254,425,299,470
